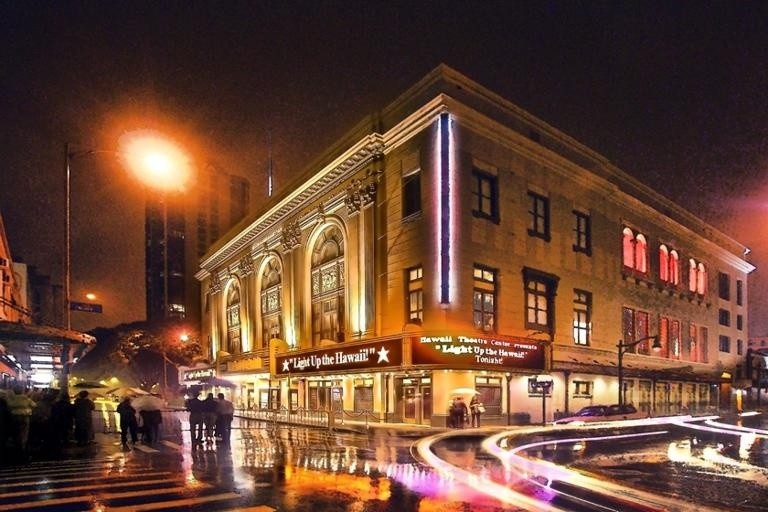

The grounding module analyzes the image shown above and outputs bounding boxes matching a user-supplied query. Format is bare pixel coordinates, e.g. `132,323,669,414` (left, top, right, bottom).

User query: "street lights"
62,125,198,388
615,334,664,405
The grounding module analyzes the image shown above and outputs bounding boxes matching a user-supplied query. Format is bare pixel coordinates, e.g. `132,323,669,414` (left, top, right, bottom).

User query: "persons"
447,396,483,428
216,392,234,440
118,397,139,451
0,383,96,448
202,392,217,440
188,391,204,443
139,410,163,444
190,438,237,493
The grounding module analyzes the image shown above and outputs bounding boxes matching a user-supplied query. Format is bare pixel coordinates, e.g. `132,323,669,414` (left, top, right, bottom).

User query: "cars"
555,404,650,425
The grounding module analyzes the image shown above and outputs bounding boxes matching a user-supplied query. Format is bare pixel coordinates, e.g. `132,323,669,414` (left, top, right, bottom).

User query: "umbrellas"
452,387,481,394
205,378,235,388
186,384,203,393
113,387,149,396
76,381,109,389
132,395,163,409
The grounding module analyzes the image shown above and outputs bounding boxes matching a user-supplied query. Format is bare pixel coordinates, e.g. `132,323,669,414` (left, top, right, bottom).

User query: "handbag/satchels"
477,407,486,414
